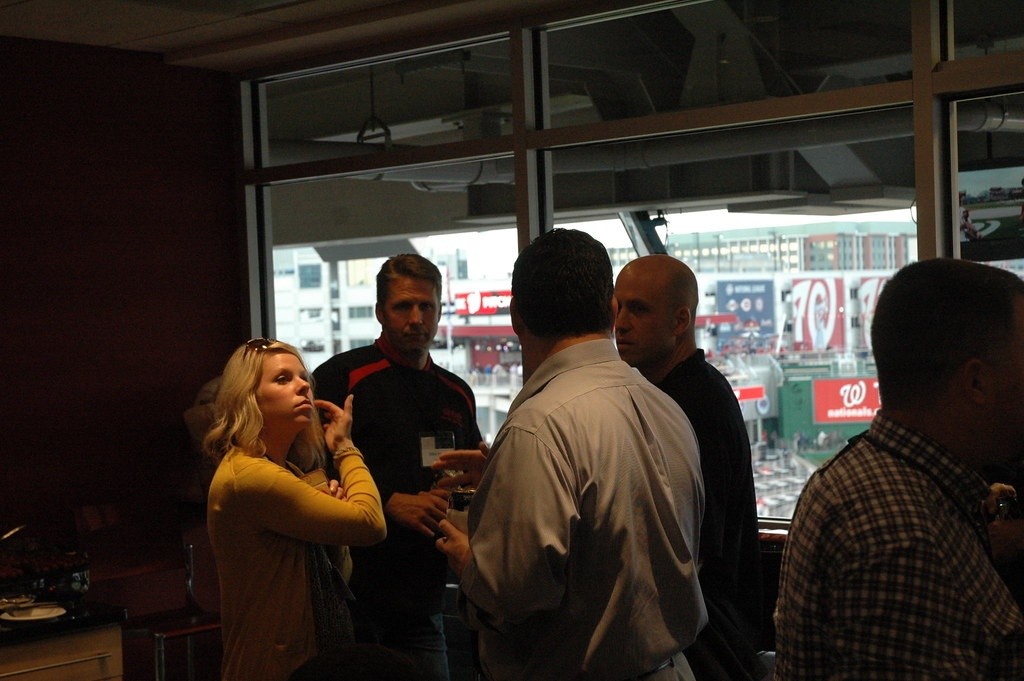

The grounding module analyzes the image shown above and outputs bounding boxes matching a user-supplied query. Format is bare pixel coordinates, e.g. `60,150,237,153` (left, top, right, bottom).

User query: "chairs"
122,524,225,681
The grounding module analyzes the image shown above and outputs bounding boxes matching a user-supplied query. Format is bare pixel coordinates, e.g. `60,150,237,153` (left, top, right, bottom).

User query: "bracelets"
333,447,364,468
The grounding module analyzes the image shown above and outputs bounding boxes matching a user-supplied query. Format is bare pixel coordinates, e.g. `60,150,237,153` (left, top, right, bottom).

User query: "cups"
427,464,473,539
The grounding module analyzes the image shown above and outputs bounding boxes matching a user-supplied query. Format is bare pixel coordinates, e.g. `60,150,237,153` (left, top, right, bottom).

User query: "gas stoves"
0,592,129,646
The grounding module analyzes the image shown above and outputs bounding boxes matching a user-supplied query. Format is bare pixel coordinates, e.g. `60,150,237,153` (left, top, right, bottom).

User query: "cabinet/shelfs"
0,602,128,681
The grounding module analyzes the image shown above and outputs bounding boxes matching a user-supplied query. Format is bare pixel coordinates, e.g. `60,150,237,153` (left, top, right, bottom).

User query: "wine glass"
444,488,477,590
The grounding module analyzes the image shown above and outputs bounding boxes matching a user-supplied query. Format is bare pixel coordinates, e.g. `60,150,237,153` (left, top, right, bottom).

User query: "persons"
772,257,1024,681
310,253,482,681
432,229,710,681
793,429,839,449
614,253,767,679
204,339,386,681
473,361,523,383
986,483,1016,514
